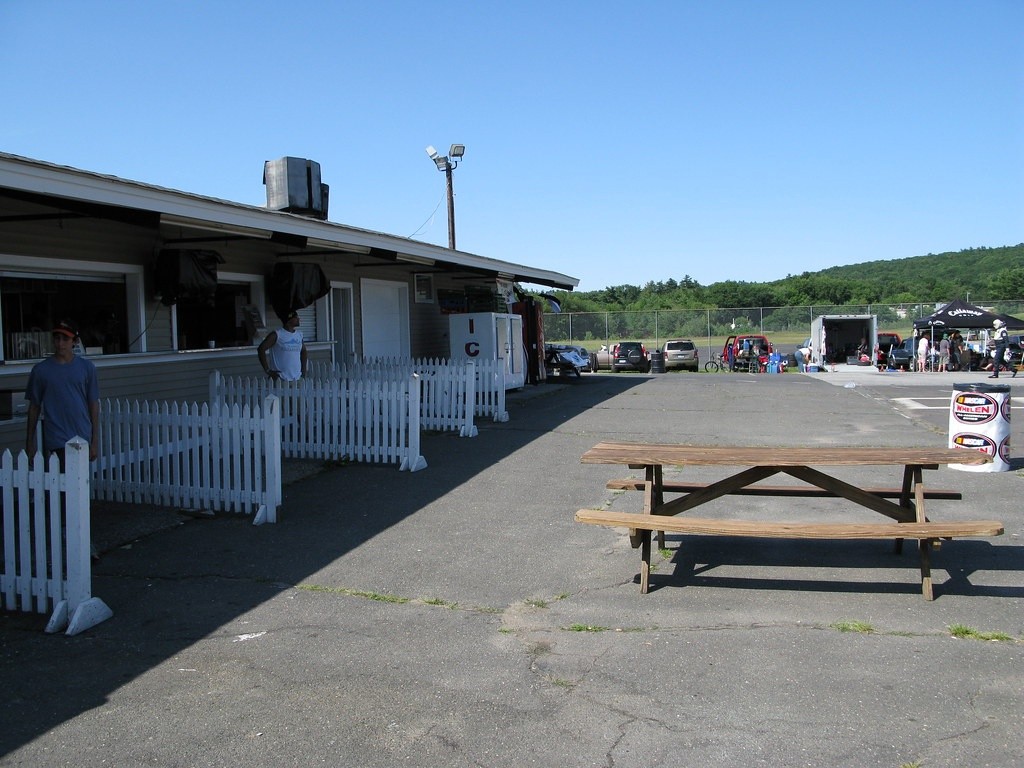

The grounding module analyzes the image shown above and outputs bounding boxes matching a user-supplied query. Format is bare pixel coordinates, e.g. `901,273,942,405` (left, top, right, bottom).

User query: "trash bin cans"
946,383,1012,474
651,354,666,373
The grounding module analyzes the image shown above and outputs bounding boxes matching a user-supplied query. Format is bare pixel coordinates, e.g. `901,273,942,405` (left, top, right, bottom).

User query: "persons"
937,333,951,373
795,346,813,373
951,330,963,371
988,318,1018,378
23,319,100,561
256,308,323,444
916,334,931,372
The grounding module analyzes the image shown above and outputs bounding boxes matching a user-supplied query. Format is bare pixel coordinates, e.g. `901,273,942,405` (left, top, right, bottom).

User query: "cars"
962,335,994,352
986,335,1024,358
544,343,591,371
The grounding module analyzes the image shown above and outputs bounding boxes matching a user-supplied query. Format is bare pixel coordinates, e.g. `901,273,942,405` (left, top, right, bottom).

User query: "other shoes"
989,375,998,378
91,544,99,560
1013,369,1017,377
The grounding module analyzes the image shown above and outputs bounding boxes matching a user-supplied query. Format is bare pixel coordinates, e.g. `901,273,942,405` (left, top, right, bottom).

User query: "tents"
912,296,1024,372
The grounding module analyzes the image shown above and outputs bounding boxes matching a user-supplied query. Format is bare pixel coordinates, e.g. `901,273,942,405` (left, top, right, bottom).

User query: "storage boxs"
810,363,819,372
857,361,871,366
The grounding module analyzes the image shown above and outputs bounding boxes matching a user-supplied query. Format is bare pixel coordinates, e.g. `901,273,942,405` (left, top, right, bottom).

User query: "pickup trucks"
596,342,617,368
797,338,812,356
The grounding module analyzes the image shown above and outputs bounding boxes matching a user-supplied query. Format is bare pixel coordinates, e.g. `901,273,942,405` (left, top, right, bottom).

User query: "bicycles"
705,352,732,374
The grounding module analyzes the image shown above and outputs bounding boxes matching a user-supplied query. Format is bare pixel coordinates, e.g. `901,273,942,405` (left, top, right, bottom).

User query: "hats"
52,320,75,338
281,311,297,324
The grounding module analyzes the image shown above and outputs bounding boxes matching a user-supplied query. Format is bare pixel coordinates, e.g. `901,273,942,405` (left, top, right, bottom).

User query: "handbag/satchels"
1004,351,1012,363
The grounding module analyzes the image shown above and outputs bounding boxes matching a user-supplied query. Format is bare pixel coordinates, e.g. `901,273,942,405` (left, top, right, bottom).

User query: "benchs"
558,358,591,370
606,478,963,509
574,508,1004,562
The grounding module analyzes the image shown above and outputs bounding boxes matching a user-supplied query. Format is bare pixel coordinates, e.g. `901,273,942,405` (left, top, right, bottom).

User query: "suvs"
876,332,902,364
887,329,958,371
610,343,649,374
722,333,773,368
655,339,699,372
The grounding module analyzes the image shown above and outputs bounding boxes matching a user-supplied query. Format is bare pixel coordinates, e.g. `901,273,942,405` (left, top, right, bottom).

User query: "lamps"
498,271,515,279
396,252,435,266
306,237,371,255
160,213,272,239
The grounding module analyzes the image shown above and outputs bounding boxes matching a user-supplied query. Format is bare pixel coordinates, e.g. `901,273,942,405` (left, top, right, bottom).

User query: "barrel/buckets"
770,364,777,373
771,353,781,372
651,353,665,374
807,365,818,372
947,383,1011,472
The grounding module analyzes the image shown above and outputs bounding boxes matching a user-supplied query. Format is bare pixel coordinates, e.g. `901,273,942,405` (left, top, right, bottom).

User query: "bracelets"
266,368,273,373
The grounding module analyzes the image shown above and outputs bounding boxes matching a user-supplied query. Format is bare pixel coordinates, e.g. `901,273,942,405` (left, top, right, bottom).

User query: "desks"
580,440,994,602
544,349,581,382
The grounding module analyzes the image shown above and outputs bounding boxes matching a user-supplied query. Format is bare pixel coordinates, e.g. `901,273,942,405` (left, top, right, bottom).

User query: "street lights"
426,145,466,249
